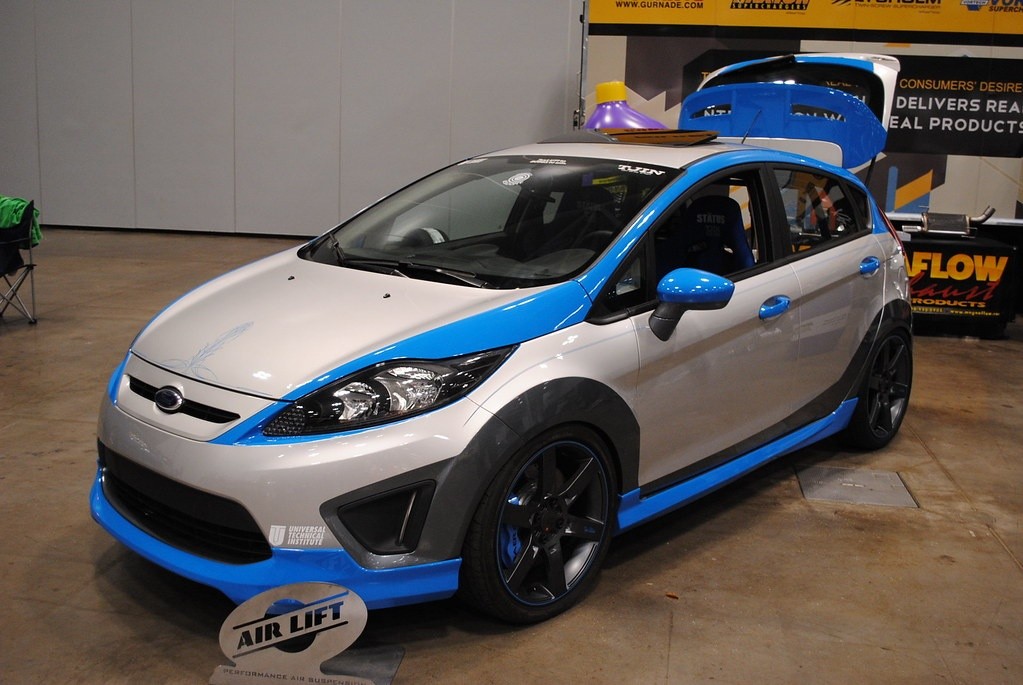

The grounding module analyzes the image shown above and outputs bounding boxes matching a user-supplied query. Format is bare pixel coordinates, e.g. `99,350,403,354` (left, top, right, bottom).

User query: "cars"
87,52,917,623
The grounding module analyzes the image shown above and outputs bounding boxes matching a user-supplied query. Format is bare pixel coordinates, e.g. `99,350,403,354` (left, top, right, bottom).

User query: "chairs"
0,195,37,325
625,195,756,294
498,187,614,263
